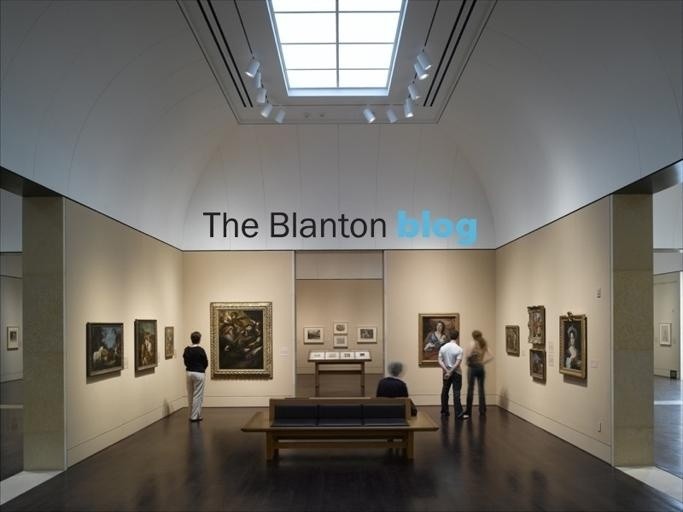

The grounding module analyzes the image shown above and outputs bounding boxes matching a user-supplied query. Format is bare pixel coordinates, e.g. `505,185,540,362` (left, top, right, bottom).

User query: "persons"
139,322,156,367
564,324,582,369
376,362,418,416
182,331,208,423
462,329,496,420
423,320,450,353
438,330,464,418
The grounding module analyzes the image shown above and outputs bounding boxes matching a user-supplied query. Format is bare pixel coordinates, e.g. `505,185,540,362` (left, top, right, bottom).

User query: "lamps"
244,59,286,125
362,53,433,123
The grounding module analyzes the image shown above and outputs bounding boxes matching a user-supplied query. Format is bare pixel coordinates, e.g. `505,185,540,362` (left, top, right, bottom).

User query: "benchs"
240,397,440,466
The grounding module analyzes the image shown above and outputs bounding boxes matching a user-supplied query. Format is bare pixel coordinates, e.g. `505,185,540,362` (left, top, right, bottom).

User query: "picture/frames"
659,323,671,346
7,326,19,350
526,305,545,345
559,311,587,380
303,324,378,360
210,302,273,379
165,327,174,357
505,325,520,357
134,319,158,372
418,313,459,366
86,322,124,377
529,349,546,381
